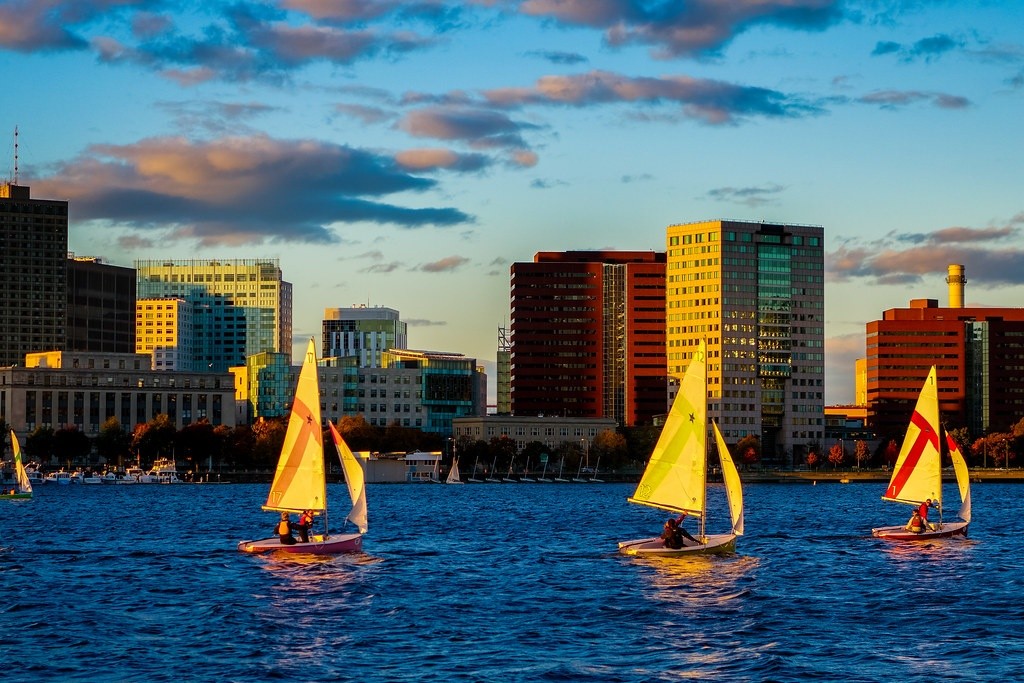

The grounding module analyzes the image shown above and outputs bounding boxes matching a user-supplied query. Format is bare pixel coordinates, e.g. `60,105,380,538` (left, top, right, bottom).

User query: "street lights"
839,438,843,472
1003,439,1008,474
581,439,588,469
854,440,859,472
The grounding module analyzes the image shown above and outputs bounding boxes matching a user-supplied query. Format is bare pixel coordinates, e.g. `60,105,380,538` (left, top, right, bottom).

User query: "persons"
905,508,936,534
917,498,932,520
273,512,299,545
2,487,8,495
664,519,706,549
187,470,192,482
296,509,314,543
10,488,15,495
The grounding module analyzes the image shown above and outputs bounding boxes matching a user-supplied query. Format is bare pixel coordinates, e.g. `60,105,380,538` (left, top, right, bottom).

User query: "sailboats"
871,365,971,541
446,456,604,484
237,336,368,555
0,429,33,498
618,333,744,556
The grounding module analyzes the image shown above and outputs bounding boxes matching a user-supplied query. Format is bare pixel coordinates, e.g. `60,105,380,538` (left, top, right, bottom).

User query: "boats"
0,457,183,484
